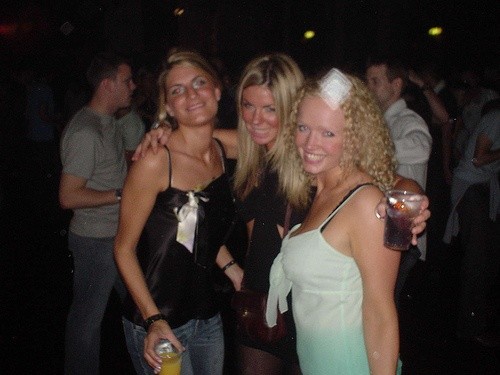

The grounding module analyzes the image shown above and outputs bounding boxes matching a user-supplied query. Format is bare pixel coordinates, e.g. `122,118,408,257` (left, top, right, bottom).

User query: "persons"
27,42,500,375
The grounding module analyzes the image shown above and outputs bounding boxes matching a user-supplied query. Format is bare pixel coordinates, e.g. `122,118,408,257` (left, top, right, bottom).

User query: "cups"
375,189,424,251
153,342,183,375
